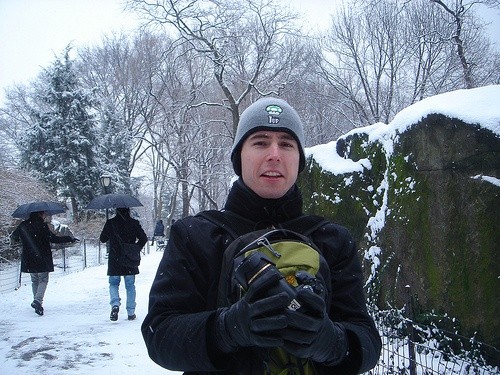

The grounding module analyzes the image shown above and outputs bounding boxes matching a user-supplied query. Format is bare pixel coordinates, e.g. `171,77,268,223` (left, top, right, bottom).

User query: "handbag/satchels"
110,220,141,263
21,222,52,259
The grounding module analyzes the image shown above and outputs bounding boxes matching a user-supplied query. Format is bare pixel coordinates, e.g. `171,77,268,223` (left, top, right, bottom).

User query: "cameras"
231,251,317,318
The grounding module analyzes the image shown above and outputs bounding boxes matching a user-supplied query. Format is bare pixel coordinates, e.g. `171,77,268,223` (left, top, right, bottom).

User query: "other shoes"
128,313,136,320
110,305,119,321
31,299,44,315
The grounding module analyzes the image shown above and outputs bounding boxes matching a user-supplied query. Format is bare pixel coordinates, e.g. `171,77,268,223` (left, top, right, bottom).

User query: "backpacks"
196,210,330,375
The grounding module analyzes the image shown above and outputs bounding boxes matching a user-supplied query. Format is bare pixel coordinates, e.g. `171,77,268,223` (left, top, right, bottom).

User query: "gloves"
214,269,290,356
277,288,349,368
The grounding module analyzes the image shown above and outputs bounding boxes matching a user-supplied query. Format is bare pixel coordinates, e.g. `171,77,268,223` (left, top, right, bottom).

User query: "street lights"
100,171,113,257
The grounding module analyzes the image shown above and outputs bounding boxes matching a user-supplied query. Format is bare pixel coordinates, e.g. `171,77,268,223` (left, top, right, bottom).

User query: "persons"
149,220,164,246
170,219,175,227
10,211,80,316
100,208,148,321
141,97,382,375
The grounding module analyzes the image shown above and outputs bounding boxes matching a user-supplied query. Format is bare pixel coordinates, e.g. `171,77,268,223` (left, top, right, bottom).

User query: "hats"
230,98,306,176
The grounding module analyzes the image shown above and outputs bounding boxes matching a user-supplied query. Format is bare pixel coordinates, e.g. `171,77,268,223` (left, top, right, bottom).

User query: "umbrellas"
85,194,144,209
11,202,66,220
